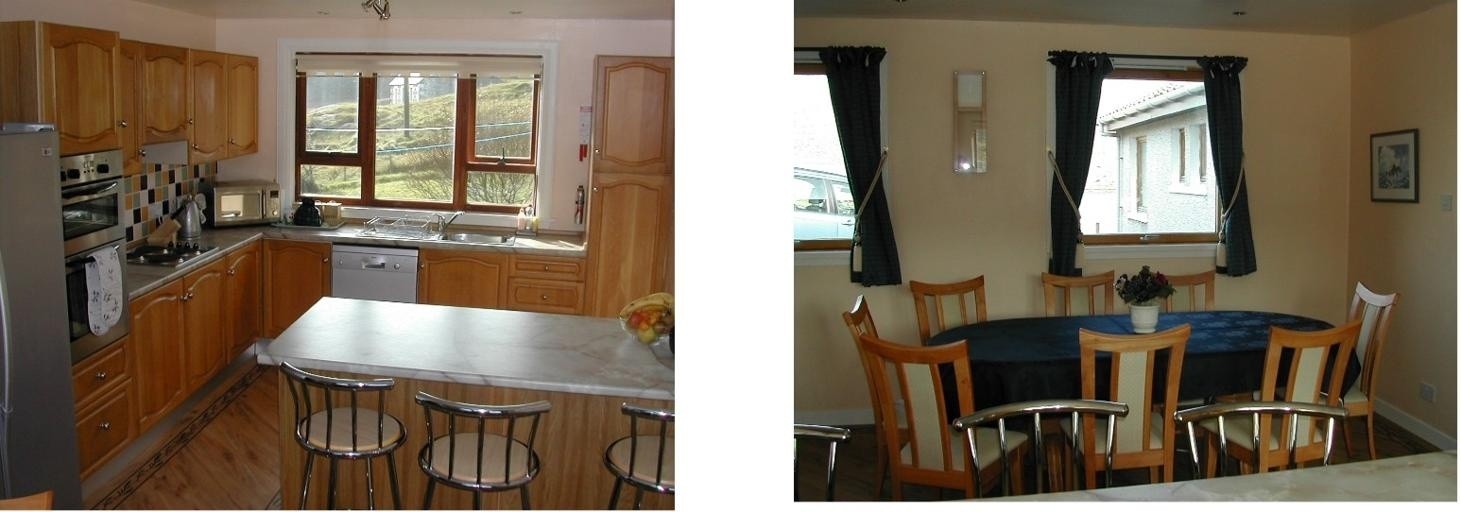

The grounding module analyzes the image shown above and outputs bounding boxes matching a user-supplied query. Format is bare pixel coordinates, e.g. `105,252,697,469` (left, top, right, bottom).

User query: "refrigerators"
0,120,82,510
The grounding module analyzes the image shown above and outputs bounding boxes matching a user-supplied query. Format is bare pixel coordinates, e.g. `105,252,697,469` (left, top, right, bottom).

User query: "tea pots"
178,194,202,240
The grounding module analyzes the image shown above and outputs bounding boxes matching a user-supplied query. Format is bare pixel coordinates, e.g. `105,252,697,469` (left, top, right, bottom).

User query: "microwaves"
212,181,281,227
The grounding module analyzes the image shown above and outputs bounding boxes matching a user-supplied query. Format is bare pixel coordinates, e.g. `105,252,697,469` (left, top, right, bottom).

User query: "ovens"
57,146,127,256
64,239,130,365
331,244,419,303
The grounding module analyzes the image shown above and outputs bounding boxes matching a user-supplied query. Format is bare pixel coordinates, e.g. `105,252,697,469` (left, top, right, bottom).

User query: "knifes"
170,206,184,219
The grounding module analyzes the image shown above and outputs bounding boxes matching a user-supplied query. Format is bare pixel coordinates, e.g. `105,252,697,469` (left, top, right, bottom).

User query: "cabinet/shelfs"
0,20,127,156
585,53,675,317
192,49,228,164
262,240,332,341
226,240,264,364
229,55,259,159
142,42,192,165
416,248,508,311
129,255,226,436
121,39,144,176
71,332,138,482
507,251,585,315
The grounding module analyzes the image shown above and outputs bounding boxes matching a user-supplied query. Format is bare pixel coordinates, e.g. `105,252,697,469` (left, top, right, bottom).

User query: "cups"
285,208,296,224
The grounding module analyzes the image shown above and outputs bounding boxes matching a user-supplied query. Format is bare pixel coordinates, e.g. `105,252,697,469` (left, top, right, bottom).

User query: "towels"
86,248,124,337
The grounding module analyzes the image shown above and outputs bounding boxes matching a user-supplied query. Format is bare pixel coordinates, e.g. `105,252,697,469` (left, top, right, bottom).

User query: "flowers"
1114,265,1177,304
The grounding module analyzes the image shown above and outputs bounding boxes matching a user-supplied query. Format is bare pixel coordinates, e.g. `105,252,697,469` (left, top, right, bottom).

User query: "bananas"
620,292,675,319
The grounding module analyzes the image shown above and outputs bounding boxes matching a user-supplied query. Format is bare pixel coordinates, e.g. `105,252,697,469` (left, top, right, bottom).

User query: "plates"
620,317,674,346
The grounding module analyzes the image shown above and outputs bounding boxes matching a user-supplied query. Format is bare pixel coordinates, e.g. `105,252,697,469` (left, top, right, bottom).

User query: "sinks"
439,229,516,246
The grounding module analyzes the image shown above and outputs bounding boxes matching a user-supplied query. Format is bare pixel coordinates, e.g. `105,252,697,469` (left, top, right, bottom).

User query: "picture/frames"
1370,129,1419,204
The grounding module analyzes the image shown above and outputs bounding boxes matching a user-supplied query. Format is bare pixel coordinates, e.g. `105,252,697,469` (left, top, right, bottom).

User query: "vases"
1131,307,1159,334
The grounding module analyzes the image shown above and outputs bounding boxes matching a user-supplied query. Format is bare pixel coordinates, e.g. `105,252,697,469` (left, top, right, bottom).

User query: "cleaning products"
517,204,538,233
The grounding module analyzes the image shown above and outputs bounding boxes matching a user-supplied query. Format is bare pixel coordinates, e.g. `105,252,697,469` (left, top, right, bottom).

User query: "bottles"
518,205,539,233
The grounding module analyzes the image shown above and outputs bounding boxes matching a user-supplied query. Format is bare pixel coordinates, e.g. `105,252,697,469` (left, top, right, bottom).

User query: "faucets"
431,210,465,233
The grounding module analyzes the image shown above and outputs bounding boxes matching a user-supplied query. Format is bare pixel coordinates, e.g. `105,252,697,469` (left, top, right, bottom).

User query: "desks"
924,309,1361,488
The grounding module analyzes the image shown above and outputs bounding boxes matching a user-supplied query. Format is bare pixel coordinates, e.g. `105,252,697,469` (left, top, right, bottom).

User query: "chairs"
1042,270,1114,434
952,399,1128,498
909,274,987,347
1149,270,1216,437
843,295,944,499
1276,280,1400,460
278,362,407,510
795,424,852,500
604,401,675,510
1198,320,1362,478
415,390,553,510
1174,405,1350,480
857,333,1032,500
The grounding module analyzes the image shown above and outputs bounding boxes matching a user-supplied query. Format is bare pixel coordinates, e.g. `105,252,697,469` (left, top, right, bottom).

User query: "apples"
637,327,654,343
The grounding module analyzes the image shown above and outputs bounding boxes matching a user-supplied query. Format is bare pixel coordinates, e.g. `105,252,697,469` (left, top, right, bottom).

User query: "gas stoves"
127,241,220,268
